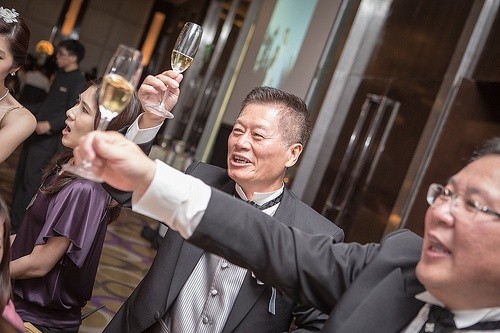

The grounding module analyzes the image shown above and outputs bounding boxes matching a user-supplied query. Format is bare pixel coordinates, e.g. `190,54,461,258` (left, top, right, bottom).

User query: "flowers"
0,6,21,25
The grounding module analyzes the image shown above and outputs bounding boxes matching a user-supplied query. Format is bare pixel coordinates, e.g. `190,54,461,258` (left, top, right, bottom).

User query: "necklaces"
0,88,9,100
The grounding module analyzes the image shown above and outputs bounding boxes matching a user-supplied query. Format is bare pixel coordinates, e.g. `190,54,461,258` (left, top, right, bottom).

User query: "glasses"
425,182,500,222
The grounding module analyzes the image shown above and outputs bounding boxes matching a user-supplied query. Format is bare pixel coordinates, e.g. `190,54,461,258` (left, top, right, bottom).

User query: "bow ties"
428,306,457,333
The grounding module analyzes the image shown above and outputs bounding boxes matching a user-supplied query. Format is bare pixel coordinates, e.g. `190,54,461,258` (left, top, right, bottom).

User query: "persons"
0,200,30,333
74,131,500,333
0,0,36,163
7,78,143,333
8,40,89,235
103,69,346,333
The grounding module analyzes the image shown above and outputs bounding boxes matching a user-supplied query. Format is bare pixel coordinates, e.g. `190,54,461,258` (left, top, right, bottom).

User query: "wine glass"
144,22,204,120
61,43,144,183
